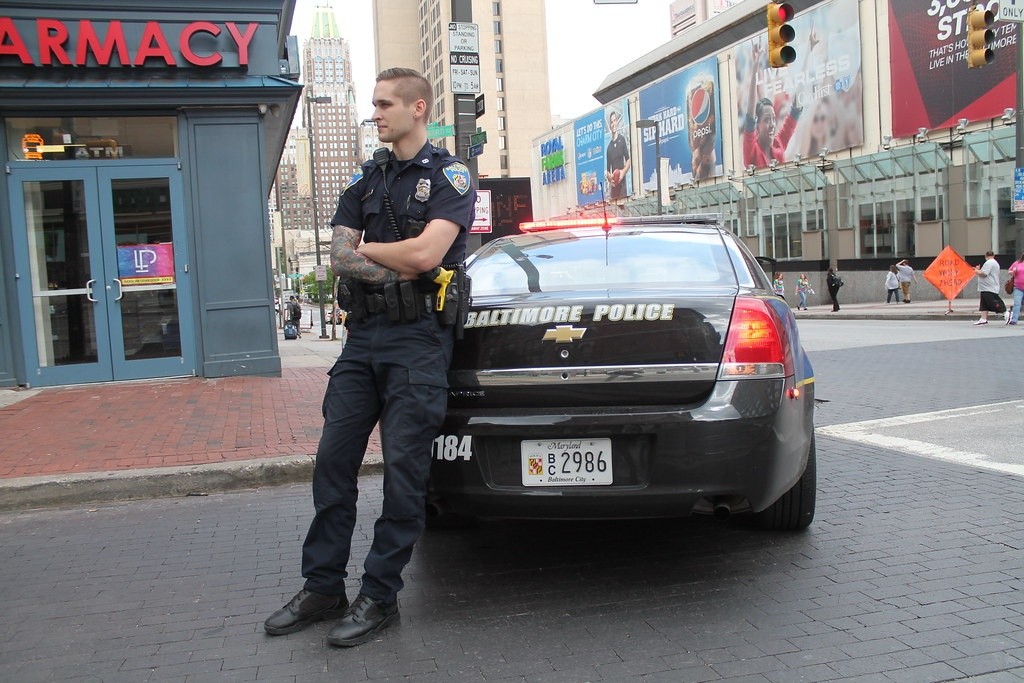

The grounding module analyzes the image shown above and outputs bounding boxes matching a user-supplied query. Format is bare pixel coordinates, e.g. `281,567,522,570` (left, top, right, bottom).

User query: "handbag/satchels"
1004,276,1014,294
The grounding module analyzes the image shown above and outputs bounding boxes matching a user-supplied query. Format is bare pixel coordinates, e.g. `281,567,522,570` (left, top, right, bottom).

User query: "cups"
686,76,716,180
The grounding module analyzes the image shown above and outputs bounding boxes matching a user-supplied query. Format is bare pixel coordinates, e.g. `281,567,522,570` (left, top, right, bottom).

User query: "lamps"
882,135,892,147
793,154,803,163
644,189,650,196
565,206,572,215
917,128,929,139
673,181,681,190
689,178,695,186
769,159,779,169
747,163,758,176
1001,106,1016,122
605,195,612,204
956,118,970,131
631,192,636,201
595,200,600,208
818,148,830,157
576,204,582,212
727,168,736,177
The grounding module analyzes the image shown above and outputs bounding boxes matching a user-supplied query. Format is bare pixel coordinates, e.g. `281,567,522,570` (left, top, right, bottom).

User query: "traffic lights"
966,10,995,69
767,4,796,68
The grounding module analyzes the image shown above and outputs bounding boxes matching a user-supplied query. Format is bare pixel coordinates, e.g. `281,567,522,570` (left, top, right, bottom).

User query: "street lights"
636,119,662,215
306,96,332,340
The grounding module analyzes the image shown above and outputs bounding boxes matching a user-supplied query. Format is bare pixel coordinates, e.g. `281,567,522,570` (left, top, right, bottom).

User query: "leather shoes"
263,587,349,636
327,593,400,648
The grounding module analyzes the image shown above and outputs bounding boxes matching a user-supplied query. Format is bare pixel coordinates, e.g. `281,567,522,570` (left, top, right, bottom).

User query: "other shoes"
1004,311,1013,325
804,308,807,310
832,309,838,312
903,299,910,303
1010,320,1016,324
974,318,988,325
797,305,800,310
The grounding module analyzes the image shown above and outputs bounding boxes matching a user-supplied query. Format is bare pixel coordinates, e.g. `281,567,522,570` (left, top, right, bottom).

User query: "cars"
425,214,816,534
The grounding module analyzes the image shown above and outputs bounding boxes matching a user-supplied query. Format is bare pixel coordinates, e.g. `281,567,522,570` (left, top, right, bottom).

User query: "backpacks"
831,274,841,288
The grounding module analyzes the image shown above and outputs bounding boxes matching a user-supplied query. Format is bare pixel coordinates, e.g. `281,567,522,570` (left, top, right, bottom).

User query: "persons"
1008,253,1024,324
884,265,900,305
974,250,1013,325
773,272,785,296
896,259,918,303
826,268,840,312
605,112,630,201
286,296,302,339
261,67,475,647
729,26,861,170
795,273,810,310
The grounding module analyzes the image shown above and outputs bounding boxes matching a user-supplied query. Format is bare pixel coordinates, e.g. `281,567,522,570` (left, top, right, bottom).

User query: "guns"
431,266,459,327
